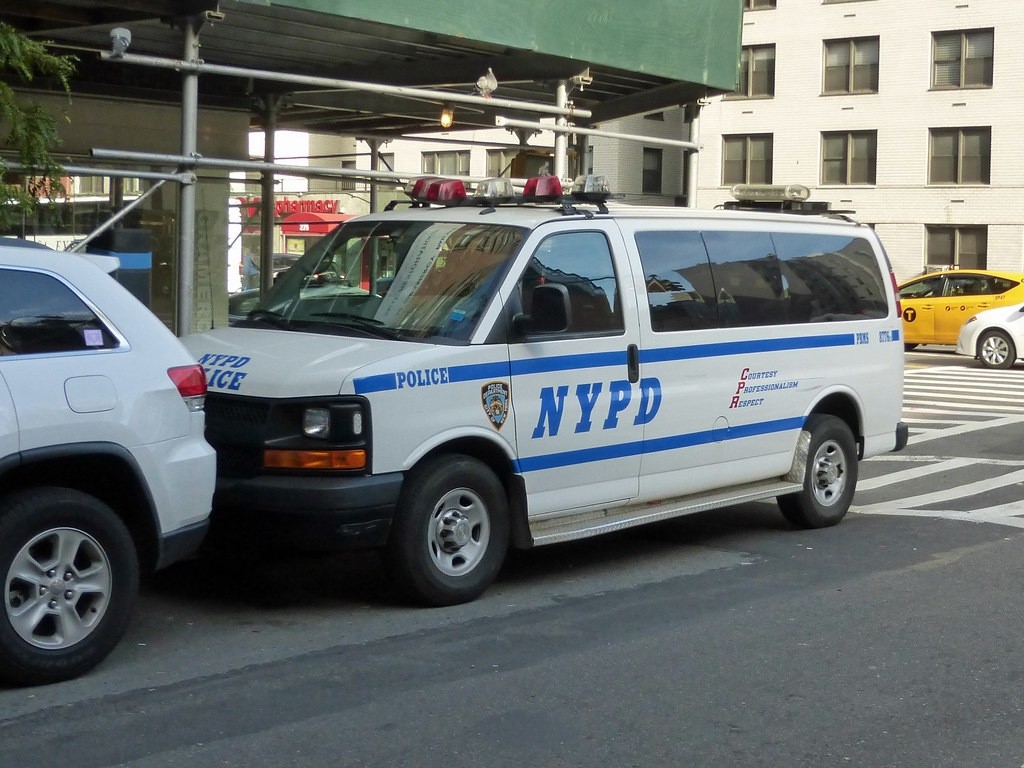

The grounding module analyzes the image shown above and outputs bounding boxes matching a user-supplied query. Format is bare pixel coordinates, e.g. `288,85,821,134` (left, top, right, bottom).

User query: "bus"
0,196,242,302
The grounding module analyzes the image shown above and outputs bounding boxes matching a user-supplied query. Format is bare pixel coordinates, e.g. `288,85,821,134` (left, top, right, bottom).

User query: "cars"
0,234,218,682
954,304,1024,369
240,252,348,288
895,267,1024,351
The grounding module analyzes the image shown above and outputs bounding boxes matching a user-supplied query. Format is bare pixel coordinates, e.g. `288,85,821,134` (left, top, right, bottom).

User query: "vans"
175,172,909,607
